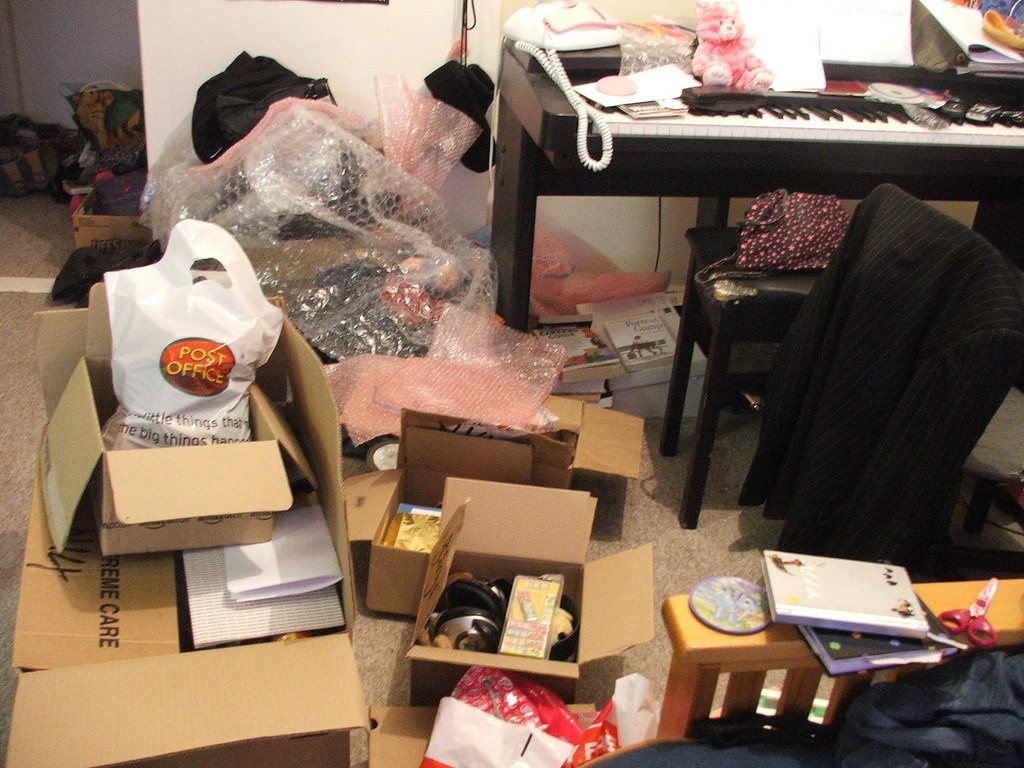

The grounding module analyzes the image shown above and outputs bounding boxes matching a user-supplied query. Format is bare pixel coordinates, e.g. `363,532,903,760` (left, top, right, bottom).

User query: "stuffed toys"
691,0,772,89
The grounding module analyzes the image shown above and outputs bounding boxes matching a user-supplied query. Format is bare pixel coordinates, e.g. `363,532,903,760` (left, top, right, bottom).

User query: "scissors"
936,577,1000,652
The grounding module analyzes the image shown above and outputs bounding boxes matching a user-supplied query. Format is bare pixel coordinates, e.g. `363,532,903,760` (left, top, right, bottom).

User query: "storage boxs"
71,194,152,250
342,425,533,616
3,296,371,768
404,474,655,707
36,281,322,560
613,377,705,418
396,395,646,491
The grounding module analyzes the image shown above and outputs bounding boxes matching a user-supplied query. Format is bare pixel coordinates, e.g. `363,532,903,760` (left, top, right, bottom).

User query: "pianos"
493,34,1024,335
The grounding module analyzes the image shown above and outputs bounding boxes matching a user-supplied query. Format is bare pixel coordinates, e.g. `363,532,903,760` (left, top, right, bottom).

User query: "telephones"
501,0,623,55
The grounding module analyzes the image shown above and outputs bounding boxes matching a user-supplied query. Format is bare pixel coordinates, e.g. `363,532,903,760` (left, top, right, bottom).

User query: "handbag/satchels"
100,219,284,451
417,665,662,768
692,190,851,284
64,81,143,148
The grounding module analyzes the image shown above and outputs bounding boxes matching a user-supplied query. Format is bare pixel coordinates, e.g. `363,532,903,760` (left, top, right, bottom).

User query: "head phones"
429,577,581,662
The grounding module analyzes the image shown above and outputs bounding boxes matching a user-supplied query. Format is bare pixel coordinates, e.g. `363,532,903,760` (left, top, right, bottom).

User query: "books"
760,551,959,677
532,295,707,407
621,101,689,117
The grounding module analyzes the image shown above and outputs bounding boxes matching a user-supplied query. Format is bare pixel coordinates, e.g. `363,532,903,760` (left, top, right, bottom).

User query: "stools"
660,226,825,530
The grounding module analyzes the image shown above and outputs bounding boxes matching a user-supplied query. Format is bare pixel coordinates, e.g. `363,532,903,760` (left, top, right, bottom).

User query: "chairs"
737,182,1024,583
